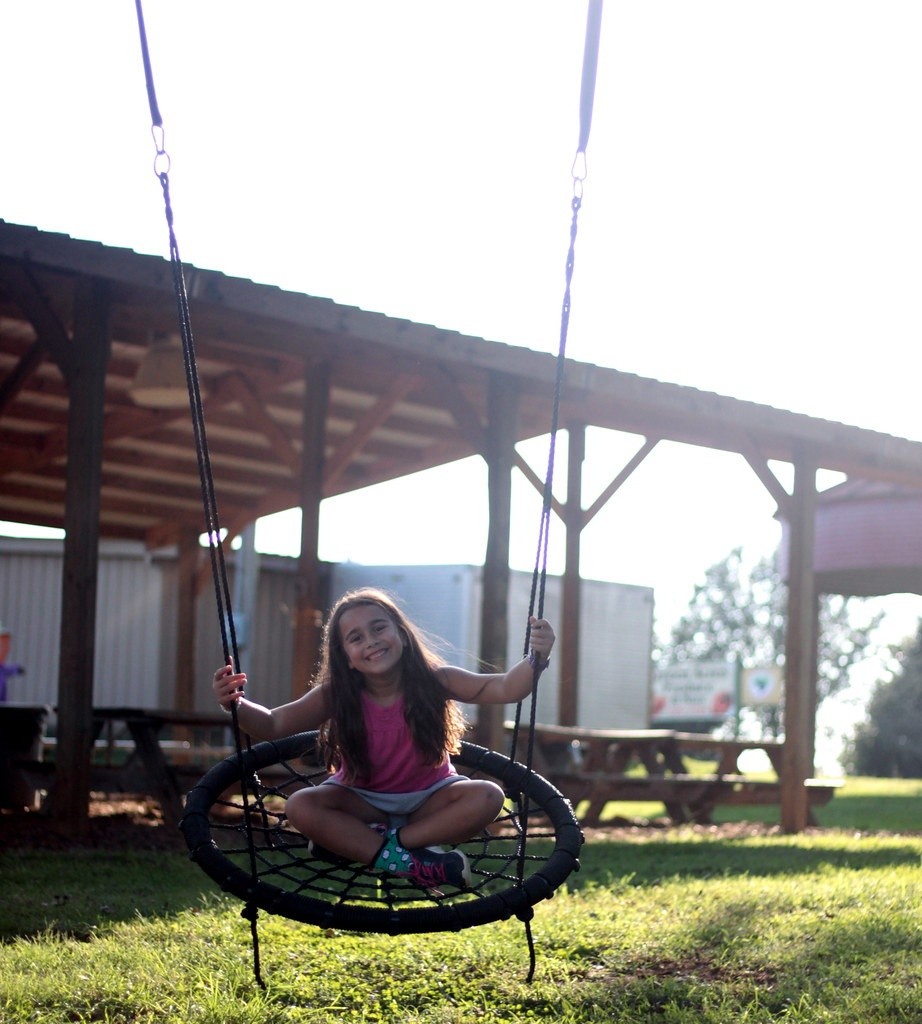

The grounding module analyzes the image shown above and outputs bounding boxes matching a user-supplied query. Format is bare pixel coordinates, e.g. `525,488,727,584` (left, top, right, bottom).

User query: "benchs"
28,741,843,830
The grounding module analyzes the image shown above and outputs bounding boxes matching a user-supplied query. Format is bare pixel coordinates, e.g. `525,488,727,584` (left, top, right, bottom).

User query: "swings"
134,2,601,933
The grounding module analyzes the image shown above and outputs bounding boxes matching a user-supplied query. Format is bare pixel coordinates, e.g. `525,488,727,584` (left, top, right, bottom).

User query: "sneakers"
308,822,388,865
398,845,473,896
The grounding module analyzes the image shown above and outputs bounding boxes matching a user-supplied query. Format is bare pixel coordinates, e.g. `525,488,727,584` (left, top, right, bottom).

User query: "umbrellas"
776,478,921,598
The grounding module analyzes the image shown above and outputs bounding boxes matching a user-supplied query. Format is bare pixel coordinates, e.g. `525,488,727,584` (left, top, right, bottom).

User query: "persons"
212,590,556,890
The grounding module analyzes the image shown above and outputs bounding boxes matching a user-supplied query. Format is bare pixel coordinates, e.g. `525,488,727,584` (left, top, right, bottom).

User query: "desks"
53,704,822,827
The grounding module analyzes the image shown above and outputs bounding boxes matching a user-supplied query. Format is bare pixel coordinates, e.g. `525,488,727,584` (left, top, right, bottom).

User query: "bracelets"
528,649,551,671
219,701,241,714
